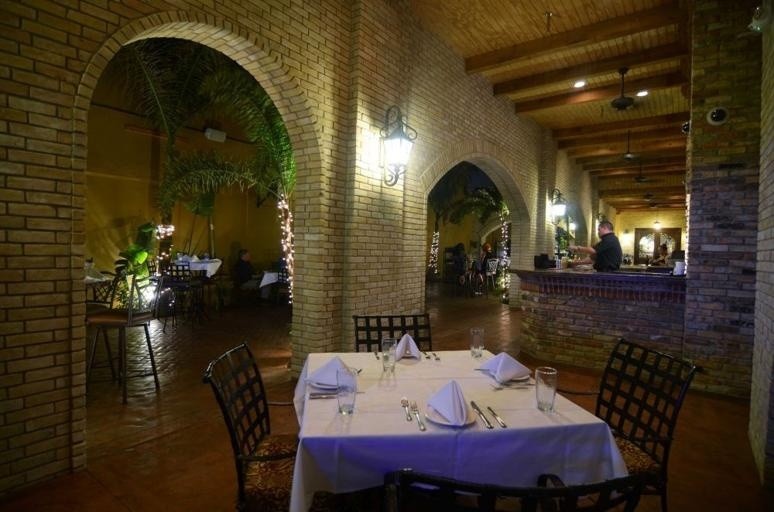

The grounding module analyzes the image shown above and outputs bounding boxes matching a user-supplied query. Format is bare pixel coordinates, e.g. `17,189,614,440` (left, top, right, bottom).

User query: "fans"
643,195,651,202
611,66,634,111
623,129,635,162
635,161,648,183
649,203,657,209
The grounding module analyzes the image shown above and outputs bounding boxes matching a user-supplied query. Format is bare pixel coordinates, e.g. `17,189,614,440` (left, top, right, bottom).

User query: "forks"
410,401,425,430
433,352,441,361
422,350,431,358
489,383,528,390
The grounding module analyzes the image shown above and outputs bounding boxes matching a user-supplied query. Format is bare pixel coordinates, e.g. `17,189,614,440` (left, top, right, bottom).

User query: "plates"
310,382,339,389
512,375,530,380
402,355,413,358
425,407,476,426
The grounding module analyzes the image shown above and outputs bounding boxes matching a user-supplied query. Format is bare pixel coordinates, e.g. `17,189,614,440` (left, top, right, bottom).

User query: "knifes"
374,350,379,360
309,389,366,397
470,401,493,428
488,406,506,427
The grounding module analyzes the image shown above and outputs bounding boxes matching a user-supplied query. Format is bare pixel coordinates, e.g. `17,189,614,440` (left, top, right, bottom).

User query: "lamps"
654,209,661,231
551,188,567,225
377,105,417,187
623,229,628,237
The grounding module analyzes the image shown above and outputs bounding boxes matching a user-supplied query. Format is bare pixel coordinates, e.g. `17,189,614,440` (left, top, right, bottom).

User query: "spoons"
401,397,412,421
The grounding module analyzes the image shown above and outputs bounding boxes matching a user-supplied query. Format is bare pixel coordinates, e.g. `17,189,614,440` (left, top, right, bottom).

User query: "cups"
336,368,358,415
470,327,489,358
535,367,558,411
675,261,685,275
382,338,398,375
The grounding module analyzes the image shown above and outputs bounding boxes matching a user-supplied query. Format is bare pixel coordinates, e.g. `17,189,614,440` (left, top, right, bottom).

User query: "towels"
396,334,421,365
307,356,356,392
473,352,533,384
428,380,470,426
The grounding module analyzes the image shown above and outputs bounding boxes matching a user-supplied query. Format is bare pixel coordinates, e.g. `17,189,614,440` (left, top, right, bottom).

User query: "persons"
233,248,253,285
471,259,484,292
483,243,496,295
650,244,671,266
567,221,623,272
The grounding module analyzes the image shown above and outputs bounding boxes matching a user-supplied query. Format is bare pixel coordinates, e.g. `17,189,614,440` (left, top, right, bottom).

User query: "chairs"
483,258,499,301
147,260,161,318
275,266,290,306
197,341,329,512
352,312,432,352
161,261,191,328
86,273,164,406
90,271,120,310
547,337,703,512
